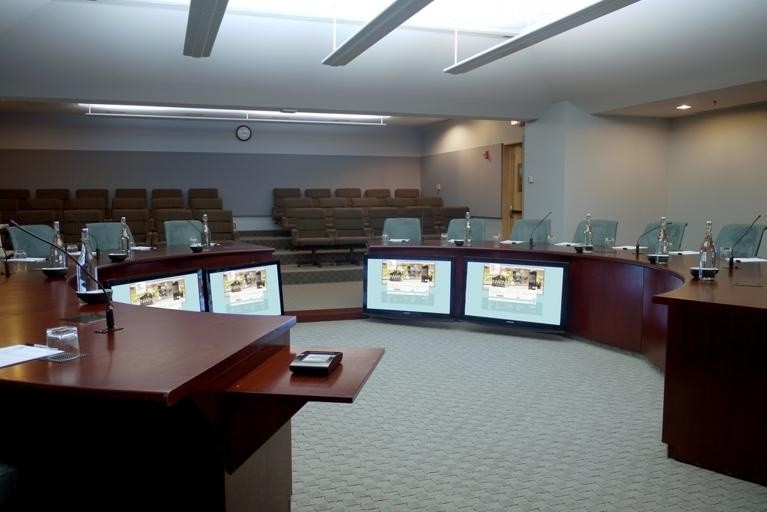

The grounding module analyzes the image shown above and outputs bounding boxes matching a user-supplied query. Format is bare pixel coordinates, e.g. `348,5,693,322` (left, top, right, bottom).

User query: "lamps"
85,103,393,126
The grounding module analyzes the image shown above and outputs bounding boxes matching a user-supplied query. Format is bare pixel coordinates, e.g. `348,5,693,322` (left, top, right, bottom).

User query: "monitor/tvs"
205,259,284,316
362,253,457,320
460,256,570,334
105,268,206,314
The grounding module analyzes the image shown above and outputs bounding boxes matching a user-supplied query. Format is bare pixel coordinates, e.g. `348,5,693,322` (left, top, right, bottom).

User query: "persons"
421,265,431,282
172,282,179,299
529,271,540,289
256,271,263,287
229,273,241,292
139,284,154,307
390,264,402,281
492,267,506,286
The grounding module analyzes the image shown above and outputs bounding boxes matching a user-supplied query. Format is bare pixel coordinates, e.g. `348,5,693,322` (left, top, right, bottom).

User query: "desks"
0,240,385,511
364,241,766,487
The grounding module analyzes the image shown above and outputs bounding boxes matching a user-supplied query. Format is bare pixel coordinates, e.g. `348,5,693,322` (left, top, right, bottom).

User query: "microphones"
678,222,688,251
529,211,552,246
9,219,123,333
188,220,218,247
728,214,761,269
636,222,674,254
78,228,100,258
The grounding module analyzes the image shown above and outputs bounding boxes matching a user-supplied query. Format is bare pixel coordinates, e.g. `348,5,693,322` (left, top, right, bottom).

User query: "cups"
45,325,81,363
440,232,448,248
13,249,28,263
604,237,614,252
719,247,732,262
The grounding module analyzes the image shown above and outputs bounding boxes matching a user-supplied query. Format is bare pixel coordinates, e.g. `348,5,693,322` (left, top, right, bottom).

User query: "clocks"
236,125,252,141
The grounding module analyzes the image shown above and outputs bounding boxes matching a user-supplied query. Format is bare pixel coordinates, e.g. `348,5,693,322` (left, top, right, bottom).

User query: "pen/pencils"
25,343,47,348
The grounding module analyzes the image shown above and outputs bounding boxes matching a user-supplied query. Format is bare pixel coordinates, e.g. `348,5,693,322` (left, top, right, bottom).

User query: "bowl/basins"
688,266,719,279
188,243,203,252
573,245,593,252
107,252,127,263
453,239,464,245
645,253,669,266
75,288,114,307
40,266,69,281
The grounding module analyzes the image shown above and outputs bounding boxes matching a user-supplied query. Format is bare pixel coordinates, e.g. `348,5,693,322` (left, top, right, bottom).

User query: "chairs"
272,188,471,265
2,188,239,259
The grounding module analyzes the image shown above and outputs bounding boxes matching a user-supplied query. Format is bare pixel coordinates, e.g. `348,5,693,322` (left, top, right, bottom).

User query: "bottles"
697,219,717,282
654,216,671,265
117,217,130,260
75,227,99,301
583,212,594,252
49,220,67,268
200,213,211,252
464,211,473,248
